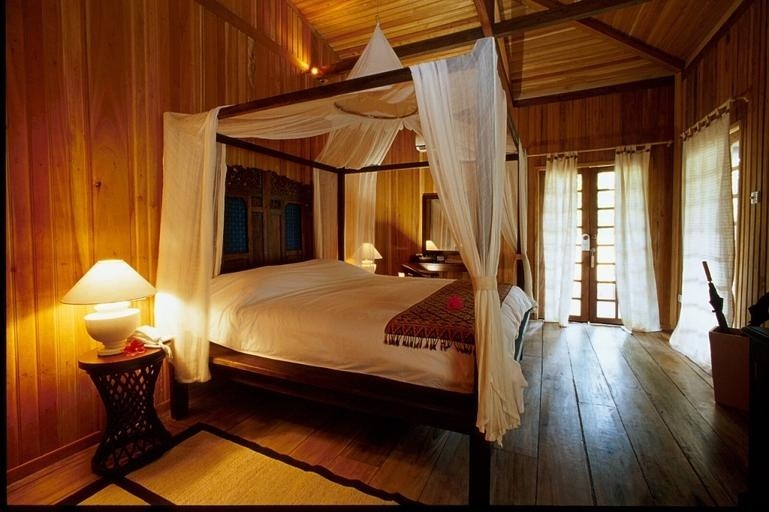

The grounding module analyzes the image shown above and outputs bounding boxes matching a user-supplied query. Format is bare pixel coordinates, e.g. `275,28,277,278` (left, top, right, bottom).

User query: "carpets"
54,421,422,507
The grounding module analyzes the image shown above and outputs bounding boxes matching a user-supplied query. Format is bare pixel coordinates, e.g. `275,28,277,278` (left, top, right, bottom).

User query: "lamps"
58,259,158,357
351,242,383,273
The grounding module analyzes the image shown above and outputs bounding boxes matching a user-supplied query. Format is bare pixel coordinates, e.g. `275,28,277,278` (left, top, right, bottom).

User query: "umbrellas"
702,261,729,330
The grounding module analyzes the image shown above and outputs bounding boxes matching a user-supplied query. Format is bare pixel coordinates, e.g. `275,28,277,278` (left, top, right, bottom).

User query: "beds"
166,49,531,506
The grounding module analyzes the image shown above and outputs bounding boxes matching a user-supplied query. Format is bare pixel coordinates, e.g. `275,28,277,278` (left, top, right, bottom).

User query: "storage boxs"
708,326,749,413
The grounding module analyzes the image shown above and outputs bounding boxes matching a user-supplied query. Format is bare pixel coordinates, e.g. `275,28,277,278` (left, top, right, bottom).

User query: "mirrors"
420,192,460,256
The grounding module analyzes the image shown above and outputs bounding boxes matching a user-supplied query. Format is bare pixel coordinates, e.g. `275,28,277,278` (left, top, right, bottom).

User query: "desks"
742,292,769,508
78,338,175,477
411,261,467,278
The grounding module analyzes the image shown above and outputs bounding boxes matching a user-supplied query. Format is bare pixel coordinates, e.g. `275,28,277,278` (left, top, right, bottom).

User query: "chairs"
401,262,440,279
